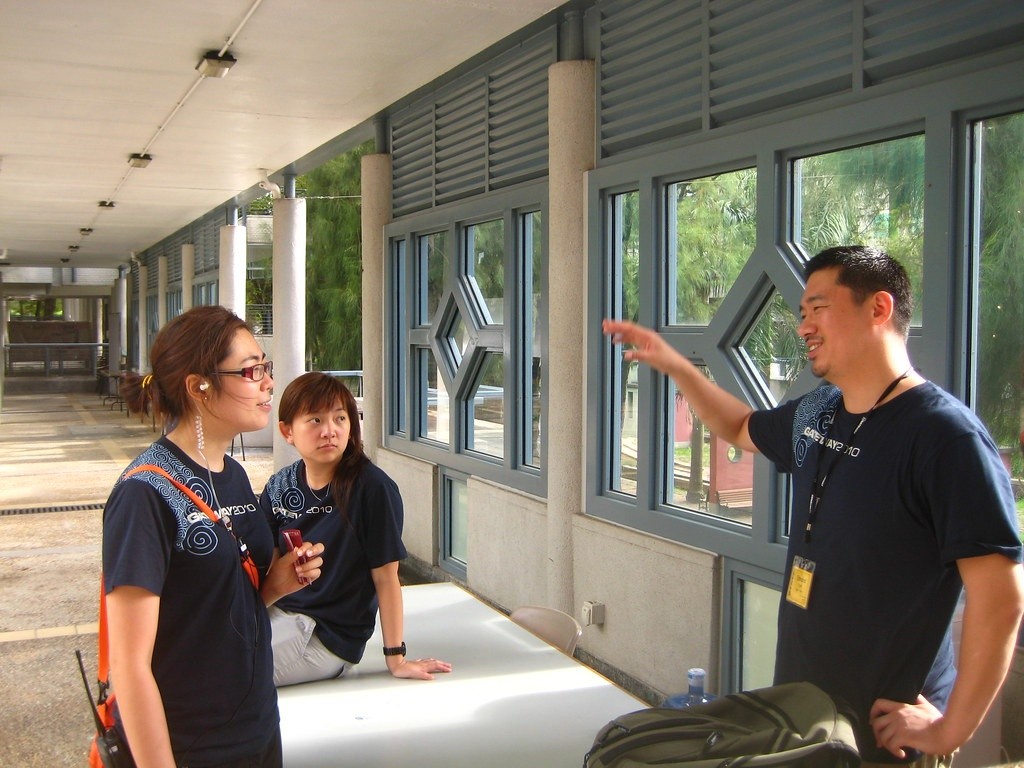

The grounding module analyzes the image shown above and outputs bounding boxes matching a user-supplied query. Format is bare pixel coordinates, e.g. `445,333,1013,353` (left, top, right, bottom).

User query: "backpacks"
585,679,859,768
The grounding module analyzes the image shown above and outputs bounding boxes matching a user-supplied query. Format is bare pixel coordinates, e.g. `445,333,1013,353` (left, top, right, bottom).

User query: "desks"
101,370,136,411
276,581,656,768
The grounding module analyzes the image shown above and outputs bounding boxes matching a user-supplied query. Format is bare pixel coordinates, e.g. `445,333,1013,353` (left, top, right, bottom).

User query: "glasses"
201,360,274,380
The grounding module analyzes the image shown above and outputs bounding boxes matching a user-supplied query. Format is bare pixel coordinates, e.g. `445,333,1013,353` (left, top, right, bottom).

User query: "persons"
102,304,324,768
259,371,452,687
585,246,1024,768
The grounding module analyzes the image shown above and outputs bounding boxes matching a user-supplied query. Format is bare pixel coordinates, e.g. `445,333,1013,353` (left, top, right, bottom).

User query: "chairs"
508,605,583,656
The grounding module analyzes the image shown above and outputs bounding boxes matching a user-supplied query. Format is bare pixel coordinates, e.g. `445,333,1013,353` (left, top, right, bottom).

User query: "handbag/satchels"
88,693,116,768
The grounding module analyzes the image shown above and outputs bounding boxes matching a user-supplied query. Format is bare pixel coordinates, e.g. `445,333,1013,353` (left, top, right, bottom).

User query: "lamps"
60,50,238,265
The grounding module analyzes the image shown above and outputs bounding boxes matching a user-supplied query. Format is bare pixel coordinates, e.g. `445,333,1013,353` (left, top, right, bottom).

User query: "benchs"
717,487,752,515
698,488,710,512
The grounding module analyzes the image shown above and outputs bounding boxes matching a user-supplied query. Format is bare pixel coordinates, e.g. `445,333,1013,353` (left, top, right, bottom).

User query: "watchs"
384,641,407,657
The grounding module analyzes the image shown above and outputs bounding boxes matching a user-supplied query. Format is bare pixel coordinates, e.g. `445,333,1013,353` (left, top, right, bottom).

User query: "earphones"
199,383,209,390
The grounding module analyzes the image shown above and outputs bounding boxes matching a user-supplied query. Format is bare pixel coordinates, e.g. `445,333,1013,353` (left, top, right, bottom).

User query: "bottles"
660,668,718,709
